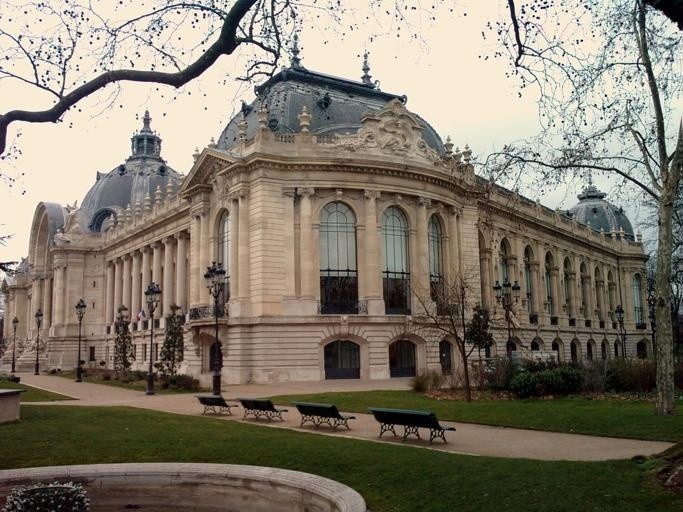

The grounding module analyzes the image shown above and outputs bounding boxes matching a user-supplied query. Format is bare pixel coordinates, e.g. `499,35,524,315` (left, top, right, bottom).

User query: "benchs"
193,395,459,446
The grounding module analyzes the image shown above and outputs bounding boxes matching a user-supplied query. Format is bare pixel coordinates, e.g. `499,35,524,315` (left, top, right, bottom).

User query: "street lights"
144,282,162,394
11,317,19,373
615,305,624,357
492,278,520,367
204,261,226,394
35,309,43,375
75,298,86,382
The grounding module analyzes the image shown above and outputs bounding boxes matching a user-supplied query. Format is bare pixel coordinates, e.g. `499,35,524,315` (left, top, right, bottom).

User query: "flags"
136,310,146,321
